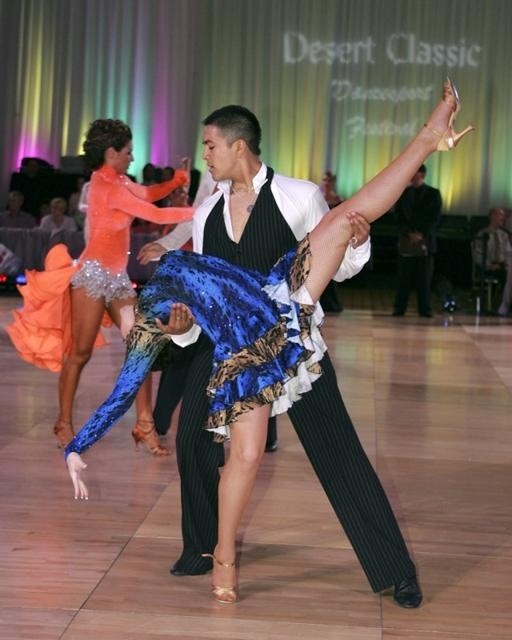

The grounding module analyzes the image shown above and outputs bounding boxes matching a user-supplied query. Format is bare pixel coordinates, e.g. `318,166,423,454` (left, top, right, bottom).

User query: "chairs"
342,208,512,317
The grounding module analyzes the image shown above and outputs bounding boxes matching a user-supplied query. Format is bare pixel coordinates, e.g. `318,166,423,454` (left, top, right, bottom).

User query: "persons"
471,207,512,316
320,171,343,209
390,165,442,317
64,74,475,603
153,104,422,609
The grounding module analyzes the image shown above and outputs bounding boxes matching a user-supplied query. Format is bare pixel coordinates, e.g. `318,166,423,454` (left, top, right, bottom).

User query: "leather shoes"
394,576,422,609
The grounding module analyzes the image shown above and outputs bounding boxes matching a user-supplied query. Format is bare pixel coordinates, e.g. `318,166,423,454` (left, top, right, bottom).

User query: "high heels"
423,76,476,151
132,419,171,456
53,419,76,448
170,553,239,603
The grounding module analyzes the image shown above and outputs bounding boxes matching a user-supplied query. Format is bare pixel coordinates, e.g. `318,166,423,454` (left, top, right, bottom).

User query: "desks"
0,229,159,285
